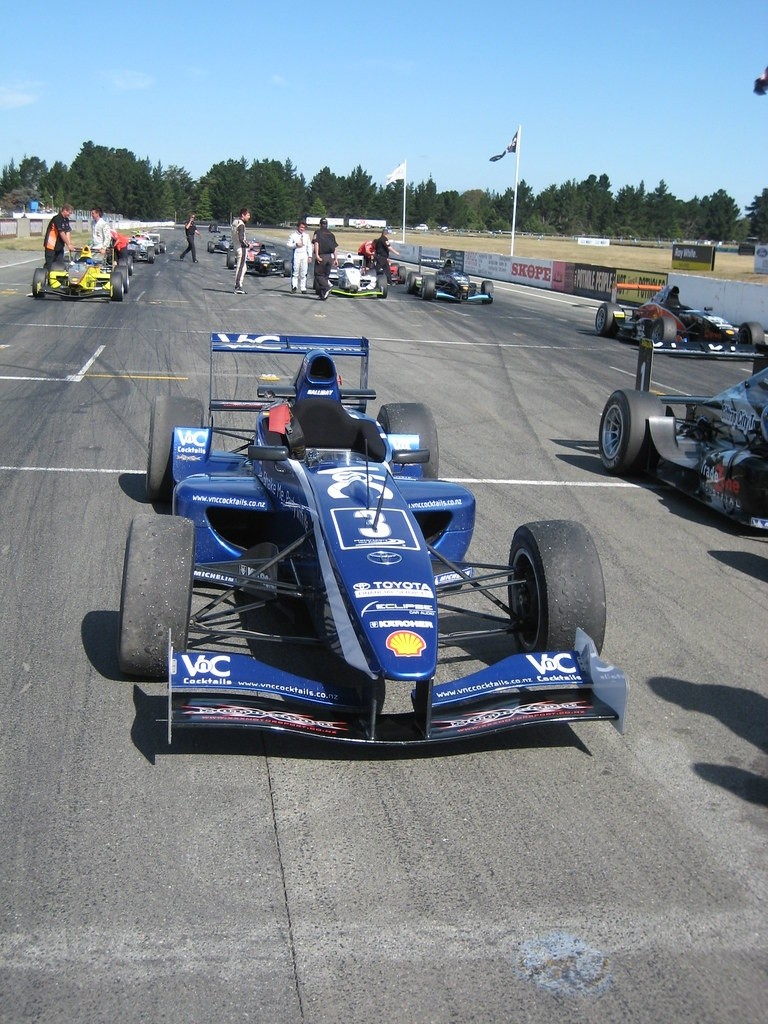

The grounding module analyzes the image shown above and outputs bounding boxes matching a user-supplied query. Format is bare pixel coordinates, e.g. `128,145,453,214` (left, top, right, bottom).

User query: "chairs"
263,399,387,461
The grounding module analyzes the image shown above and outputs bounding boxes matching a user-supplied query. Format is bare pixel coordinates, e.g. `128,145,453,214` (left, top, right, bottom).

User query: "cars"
440,226,448,232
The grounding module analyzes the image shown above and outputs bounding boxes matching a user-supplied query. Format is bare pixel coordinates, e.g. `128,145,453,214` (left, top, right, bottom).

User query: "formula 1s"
227,243,292,278
406,256,495,304
595,280,768,360
322,252,407,300
597,337,768,533
206,235,238,253
115,329,629,749
30,228,168,302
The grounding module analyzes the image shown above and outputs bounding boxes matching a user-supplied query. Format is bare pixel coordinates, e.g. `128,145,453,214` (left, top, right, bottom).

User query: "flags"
489,131,518,162
386,161,406,187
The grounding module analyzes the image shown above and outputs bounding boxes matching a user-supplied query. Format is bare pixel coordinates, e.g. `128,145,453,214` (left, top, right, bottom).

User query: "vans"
414,223,428,231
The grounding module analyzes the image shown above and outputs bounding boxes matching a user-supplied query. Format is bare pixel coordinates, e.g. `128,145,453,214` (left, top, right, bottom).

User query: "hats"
320,218,327,224
382,229,389,234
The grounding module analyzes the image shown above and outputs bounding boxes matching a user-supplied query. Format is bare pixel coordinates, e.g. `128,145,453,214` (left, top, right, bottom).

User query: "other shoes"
324,288,332,301
233,289,247,294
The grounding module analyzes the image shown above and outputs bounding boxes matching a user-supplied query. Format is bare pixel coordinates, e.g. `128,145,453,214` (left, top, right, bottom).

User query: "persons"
311,217,340,301
230,208,254,295
90,207,111,254
286,220,313,294
180,213,202,264
44,204,75,270
359,229,400,287
